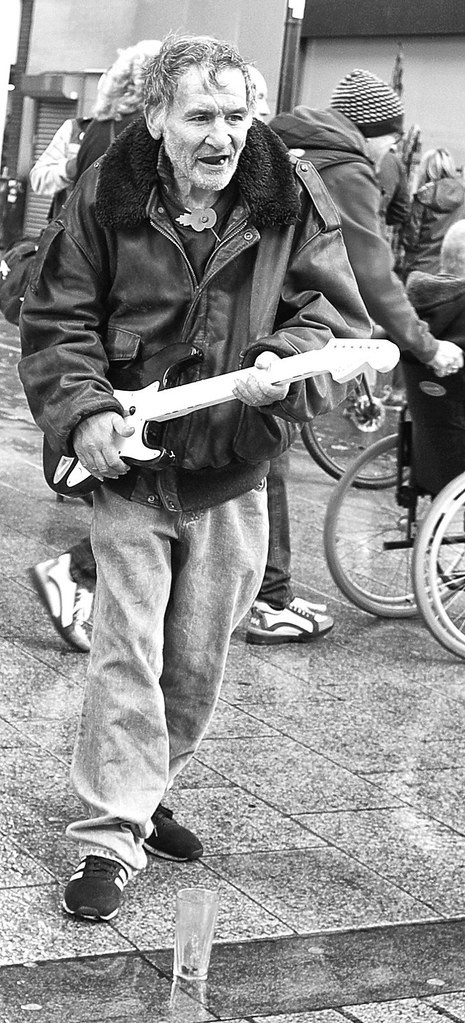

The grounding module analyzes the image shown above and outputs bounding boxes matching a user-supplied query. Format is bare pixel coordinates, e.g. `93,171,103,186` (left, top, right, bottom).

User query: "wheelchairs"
323,333,465,663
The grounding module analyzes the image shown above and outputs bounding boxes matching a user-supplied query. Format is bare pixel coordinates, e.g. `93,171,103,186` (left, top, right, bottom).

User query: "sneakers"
142,802,203,862
62,855,142,922
246,597,335,645
29,553,95,651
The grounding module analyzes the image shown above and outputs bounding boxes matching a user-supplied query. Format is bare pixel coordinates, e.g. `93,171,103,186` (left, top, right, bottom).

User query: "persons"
373,131,411,226
17,36,373,921
27,40,164,225
23,68,464,652
401,147,465,276
245,64,271,120
402,219,465,344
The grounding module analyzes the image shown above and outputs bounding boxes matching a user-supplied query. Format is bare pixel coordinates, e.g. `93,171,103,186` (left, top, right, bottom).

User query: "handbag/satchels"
0,229,46,326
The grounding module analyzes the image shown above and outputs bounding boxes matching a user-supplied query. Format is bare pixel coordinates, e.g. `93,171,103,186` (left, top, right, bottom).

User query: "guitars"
40,335,400,498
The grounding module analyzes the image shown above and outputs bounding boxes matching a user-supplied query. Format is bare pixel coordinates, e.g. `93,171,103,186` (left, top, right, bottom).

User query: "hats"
329,69,404,138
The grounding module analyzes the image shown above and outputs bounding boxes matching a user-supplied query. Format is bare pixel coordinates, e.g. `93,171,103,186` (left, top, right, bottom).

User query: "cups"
173,888,220,979
